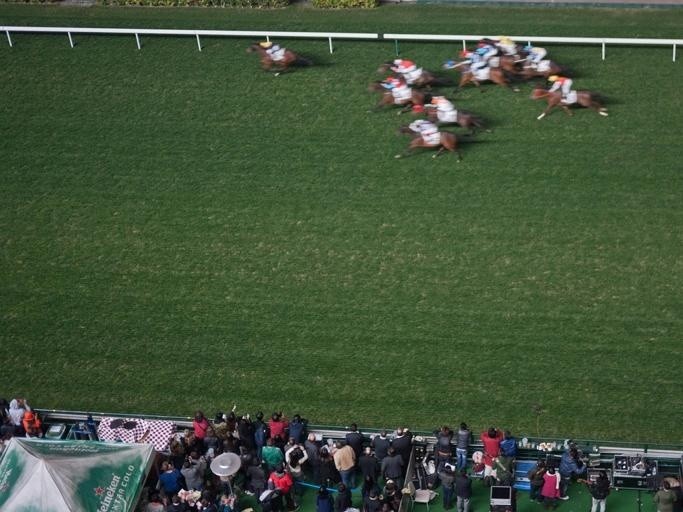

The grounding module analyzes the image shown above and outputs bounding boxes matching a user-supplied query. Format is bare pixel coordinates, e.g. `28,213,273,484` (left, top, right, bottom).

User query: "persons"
480,429,503,487
454,469,472,512
500,431,516,486
527,459,547,504
652,481,678,512
422,454,438,490
260,41,287,62
433,426,453,468
588,471,610,512
559,449,586,500
145,412,412,512
0,398,43,444
638,456,650,475
541,467,561,511
456,423,472,472
380,39,573,142
438,466,456,509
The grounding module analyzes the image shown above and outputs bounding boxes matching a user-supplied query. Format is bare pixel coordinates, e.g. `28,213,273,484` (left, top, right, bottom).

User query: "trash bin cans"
490,486,513,512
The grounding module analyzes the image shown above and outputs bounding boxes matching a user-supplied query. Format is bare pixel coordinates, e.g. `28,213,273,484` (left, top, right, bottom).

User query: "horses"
528,82,626,120
439,38,579,94
394,124,476,163
376,61,458,92
422,107,494,136
364,80,434,116
244,42,316,75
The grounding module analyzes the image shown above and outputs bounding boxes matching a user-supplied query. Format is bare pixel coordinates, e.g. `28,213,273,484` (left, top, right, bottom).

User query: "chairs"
410,487,441,512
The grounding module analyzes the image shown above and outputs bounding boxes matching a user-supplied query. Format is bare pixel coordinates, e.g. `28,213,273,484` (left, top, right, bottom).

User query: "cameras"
184,455,189,462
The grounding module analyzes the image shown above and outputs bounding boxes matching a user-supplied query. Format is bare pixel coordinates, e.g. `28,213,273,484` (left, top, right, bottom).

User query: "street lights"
209,452,240,497
